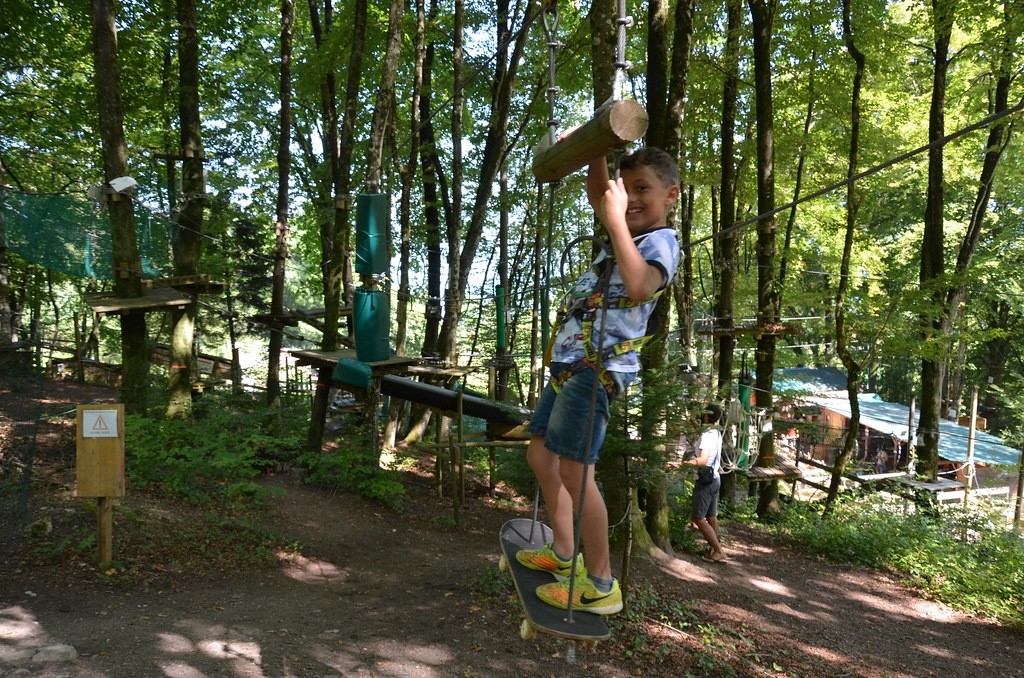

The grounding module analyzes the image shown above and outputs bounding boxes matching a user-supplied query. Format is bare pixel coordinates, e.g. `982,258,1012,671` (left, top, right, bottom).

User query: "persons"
516,146,680,616
665,404,727,564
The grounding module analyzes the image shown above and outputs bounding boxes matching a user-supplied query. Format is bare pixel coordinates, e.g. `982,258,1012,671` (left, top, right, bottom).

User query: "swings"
498,0,688,654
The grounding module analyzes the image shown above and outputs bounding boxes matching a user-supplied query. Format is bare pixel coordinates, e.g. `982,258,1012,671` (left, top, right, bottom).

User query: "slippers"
702,555,727,564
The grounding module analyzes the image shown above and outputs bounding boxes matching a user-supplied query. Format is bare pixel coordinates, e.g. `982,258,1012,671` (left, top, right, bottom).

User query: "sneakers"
516,541,585,582
536,568,623,614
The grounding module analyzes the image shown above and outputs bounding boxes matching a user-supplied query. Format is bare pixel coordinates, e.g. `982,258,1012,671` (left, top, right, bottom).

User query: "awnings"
800,392,1021,466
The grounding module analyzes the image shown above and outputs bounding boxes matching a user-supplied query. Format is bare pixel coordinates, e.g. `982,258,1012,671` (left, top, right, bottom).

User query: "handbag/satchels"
696,466,713,486
